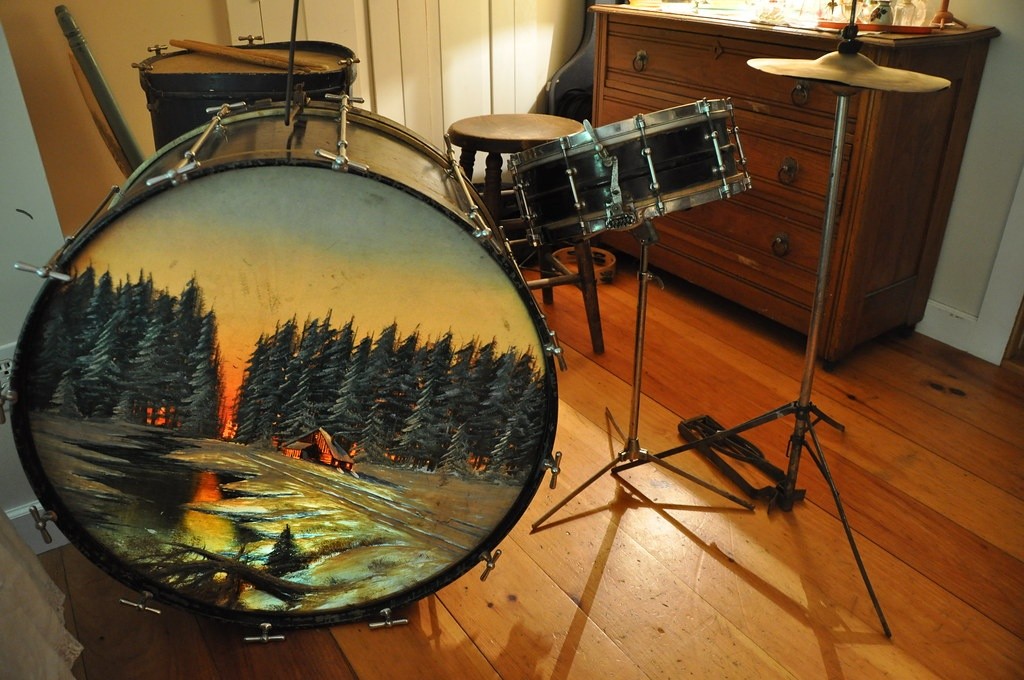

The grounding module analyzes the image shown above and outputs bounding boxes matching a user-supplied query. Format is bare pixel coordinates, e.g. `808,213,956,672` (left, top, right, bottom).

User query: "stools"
447,113,603,355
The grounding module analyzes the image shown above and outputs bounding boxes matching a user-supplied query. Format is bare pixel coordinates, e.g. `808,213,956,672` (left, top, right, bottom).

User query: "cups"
842,3,863,23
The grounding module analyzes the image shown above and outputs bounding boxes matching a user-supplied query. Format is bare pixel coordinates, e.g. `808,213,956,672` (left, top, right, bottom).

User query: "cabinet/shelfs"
587,5,1002,373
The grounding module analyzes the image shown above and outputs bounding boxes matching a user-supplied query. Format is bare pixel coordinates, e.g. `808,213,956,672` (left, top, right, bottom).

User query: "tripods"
531,222,756,532
611,91,895,639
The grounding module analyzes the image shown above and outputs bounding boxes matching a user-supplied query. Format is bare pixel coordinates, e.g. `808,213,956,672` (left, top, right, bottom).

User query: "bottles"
869,0,893,25
911,0,926,27
892,0,916,27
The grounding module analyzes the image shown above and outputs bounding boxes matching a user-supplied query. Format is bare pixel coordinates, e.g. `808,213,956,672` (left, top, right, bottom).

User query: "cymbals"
747,47,952,95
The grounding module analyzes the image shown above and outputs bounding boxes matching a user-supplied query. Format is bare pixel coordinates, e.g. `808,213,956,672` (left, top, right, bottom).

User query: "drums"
506,97,755,251
8,101,567,642
138,40,358,151
